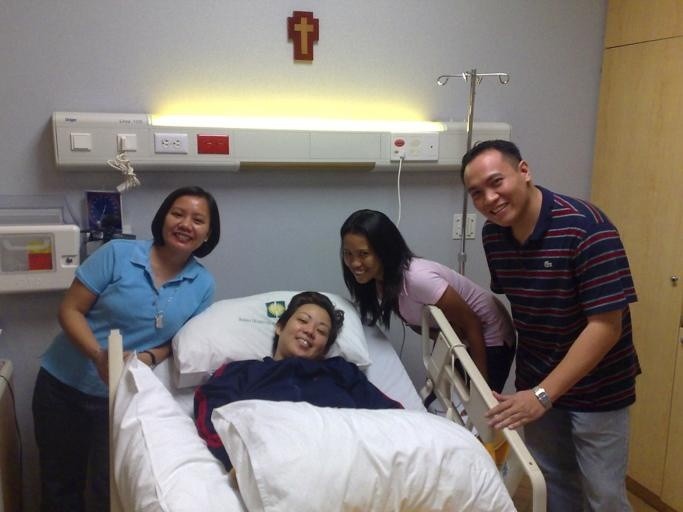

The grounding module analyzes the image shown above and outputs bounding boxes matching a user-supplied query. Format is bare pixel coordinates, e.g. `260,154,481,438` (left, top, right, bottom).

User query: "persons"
340,209,516,395
194,292,404,489
460,140,642,512
32,187,220,512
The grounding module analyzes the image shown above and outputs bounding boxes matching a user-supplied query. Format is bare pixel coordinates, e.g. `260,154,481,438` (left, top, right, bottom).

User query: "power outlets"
153,133,188,154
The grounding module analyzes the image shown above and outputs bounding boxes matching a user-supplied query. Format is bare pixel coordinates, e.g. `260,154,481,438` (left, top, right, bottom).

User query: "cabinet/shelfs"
1,359,24,510
604,0,682,49
586,36,682,512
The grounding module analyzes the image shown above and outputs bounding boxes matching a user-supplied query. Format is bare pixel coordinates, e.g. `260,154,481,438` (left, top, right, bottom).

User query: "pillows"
107,347,244,511
208,397,520,511
169,289,373,390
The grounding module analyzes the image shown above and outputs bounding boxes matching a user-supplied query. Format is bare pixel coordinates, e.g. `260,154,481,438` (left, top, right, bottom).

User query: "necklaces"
149,281,181,329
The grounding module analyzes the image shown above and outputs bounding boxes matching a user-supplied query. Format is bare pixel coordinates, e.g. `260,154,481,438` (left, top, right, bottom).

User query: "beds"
104,289,551,511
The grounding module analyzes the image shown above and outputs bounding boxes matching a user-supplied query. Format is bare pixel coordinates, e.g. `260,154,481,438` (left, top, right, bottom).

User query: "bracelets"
138,350,156,365
533,386,553,410
93,348,102,362
